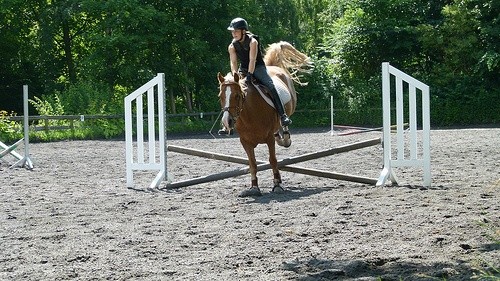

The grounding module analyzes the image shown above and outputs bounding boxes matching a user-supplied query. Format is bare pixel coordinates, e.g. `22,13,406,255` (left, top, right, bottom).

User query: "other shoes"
218,127,233,135
281,115,292,126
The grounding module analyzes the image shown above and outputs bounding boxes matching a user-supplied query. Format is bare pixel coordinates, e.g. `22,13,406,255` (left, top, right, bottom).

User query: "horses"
217,40,315,192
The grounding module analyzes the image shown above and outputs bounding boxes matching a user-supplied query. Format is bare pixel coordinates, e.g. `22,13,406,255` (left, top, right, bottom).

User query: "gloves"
246,72,254,84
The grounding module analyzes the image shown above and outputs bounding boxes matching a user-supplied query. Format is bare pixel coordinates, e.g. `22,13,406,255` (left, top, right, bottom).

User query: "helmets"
227,17,248,31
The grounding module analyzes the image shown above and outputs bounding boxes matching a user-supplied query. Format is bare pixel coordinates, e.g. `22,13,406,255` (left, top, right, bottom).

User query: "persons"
218,18,292,135
